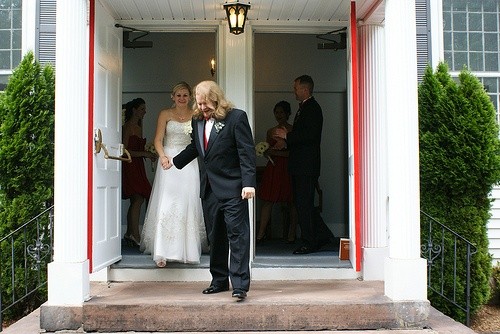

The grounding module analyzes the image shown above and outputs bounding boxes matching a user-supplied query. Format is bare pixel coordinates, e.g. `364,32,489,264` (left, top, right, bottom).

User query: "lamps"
223,0,251,35
210,59,215,76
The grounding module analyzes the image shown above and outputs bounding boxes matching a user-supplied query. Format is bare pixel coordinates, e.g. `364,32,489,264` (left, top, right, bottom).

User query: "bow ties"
204,114,216,122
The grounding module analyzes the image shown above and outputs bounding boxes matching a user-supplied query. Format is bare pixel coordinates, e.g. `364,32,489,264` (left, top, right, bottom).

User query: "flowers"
146,141,158,171
255,141,275,167
215,122,225,133
183,121,192,135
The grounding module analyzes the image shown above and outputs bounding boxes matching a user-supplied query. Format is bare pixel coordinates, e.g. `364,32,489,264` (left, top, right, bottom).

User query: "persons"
163,81,257,299
140,81,209,267
122,98,157,247
255,101,299,245
273,75,337,255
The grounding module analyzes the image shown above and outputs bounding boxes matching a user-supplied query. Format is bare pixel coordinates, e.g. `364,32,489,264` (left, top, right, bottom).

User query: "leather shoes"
232,290,247,298
203,285,229,294
293,245,313,254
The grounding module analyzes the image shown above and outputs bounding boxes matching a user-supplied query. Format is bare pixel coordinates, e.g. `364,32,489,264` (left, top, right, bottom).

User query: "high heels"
124,233,129,246
288,232,296,244
256,234,264,244
128,234,141,247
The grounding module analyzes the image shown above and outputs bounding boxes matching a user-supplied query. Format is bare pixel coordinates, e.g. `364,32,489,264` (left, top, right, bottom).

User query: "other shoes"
155,258,166,268
